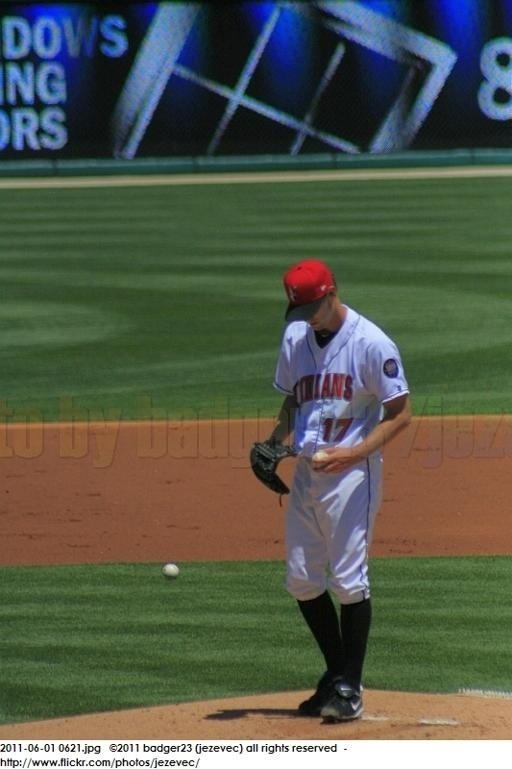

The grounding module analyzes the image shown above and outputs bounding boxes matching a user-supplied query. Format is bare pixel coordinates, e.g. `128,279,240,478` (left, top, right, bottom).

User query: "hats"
283,260,336,320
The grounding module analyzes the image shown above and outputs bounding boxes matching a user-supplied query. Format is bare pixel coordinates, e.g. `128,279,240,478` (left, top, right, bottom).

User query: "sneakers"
298,670,366,721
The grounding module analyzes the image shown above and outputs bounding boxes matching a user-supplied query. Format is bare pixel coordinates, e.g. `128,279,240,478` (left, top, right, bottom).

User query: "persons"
269,258,412,724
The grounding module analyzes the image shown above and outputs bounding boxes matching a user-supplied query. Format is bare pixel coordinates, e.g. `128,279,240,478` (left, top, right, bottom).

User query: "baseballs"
311,451,329,470
161,563,178,579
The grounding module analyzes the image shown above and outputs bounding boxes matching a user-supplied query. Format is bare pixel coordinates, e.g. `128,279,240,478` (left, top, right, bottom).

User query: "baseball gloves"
250,436,298,506
